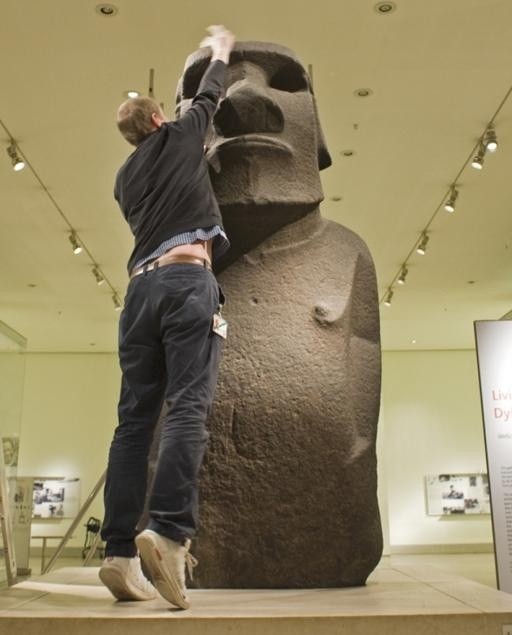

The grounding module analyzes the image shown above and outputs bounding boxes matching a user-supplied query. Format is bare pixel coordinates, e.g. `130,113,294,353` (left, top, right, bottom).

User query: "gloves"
199,24,236,66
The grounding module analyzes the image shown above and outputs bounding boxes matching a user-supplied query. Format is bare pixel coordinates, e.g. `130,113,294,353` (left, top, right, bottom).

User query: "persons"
2,438,16,466
98,21,235,611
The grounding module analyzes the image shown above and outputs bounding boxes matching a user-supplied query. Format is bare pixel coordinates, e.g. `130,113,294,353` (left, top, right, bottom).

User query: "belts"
130,254,215,278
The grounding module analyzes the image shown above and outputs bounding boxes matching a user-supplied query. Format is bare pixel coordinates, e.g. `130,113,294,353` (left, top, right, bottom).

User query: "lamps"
7,138,122,313
382,123,500,308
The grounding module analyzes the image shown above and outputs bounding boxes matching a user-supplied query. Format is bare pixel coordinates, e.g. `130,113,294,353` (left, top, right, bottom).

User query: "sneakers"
99,553,157,601
134,530,199,610
